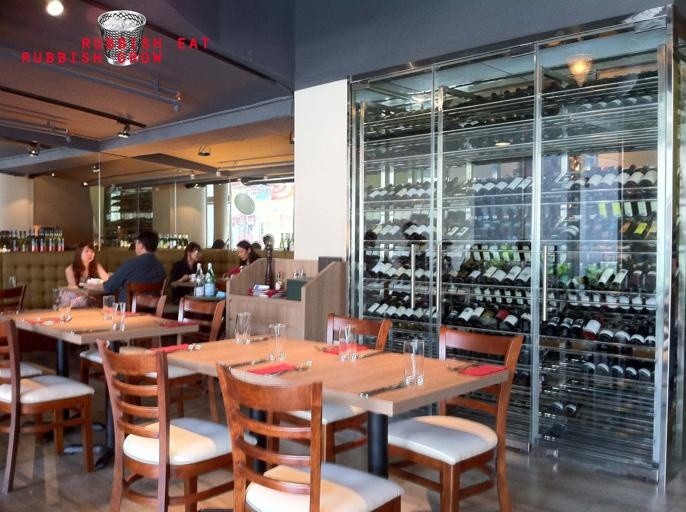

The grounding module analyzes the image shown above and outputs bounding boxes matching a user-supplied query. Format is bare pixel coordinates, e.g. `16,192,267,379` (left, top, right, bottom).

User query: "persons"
85,230,167,310
210,238,226,250
64,240,111,290
171,241,203,305
236,241,263,271
264,234,274,250
252,243,260,249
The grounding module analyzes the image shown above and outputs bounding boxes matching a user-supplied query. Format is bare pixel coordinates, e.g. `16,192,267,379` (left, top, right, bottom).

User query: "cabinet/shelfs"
536,45,676,483
434,44,537,454
359,81,436,357
103,185,154,245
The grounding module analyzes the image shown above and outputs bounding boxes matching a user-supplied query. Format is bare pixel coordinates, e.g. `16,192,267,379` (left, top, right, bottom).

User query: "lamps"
197,143,212,157
29,141,42,158
564,32,594,90
117,122,131,139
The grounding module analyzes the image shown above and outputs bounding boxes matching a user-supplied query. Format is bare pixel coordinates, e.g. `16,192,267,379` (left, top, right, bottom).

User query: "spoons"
263,361,312,377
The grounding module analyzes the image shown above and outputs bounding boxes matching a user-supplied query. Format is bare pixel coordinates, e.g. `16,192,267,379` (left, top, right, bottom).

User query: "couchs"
0,250,109,310
105,248,228,298
228,251,294,272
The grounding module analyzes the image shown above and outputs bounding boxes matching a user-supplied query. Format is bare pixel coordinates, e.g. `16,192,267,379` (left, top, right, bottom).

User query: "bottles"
192,262,216,298
365,163,657,241
363,71,660,157
1,229,64,253
356,240,656,443
273,268,305,291
119,232,189,251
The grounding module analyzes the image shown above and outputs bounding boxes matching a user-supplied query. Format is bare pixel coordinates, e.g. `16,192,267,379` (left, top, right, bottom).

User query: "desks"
225,257,347,343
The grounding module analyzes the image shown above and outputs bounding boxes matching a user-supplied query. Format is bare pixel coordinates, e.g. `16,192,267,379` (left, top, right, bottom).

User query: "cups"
50,286,125,332
337,324,358,362
400,336,424,385
268,324,288,362
234,312,252,345
